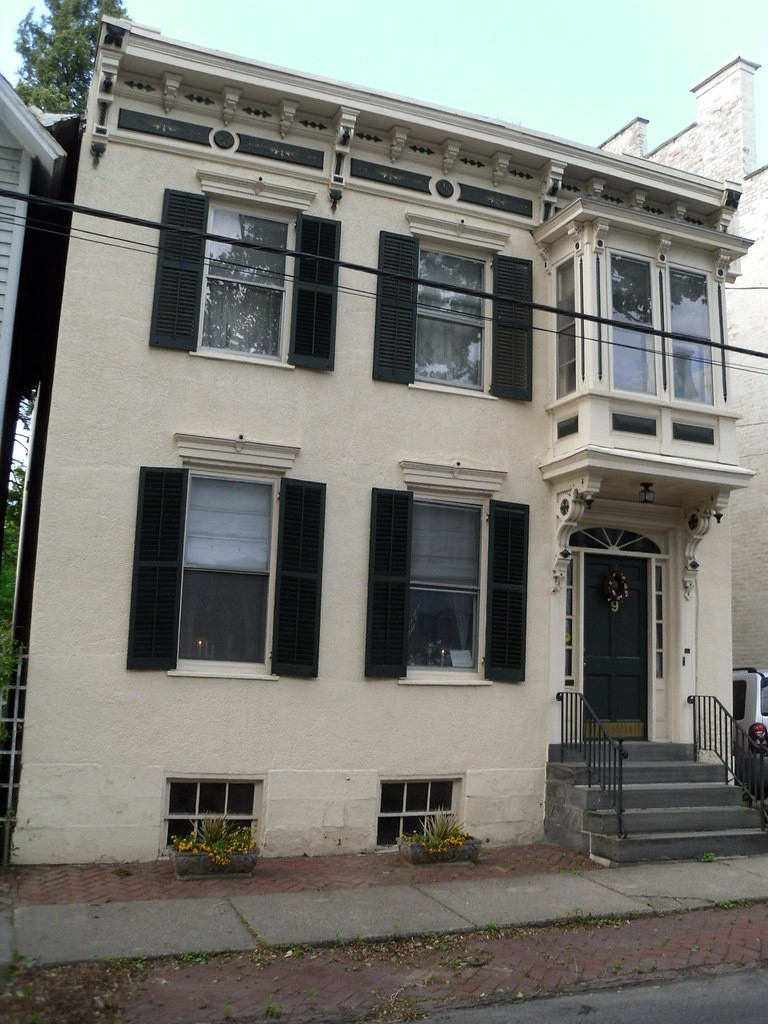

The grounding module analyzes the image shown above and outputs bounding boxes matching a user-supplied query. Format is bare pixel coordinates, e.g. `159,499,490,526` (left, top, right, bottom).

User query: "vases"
396,835,482,863
167,843,261,877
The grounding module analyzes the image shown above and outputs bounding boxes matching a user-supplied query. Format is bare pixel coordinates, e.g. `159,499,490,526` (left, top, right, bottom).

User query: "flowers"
401,804,473,854
607,570,628,603
171,810,259,865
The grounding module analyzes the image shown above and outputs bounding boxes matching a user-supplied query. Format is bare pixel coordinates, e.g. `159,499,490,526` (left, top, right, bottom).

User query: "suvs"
733,667,768,762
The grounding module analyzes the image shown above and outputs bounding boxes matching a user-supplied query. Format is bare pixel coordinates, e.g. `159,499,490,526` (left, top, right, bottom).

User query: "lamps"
639,482,654,503
